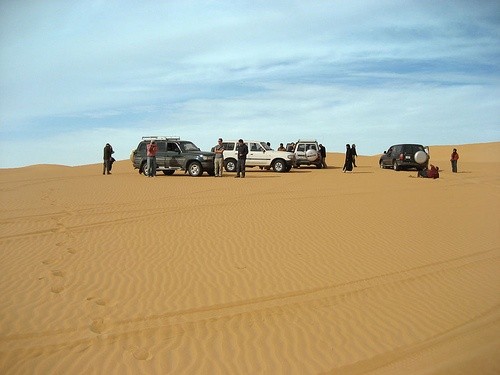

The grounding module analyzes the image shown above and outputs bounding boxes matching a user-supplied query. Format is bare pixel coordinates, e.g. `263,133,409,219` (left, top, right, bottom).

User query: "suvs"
130,136,216,177
211,140,297,173
379,144,430,171
285,139,323,169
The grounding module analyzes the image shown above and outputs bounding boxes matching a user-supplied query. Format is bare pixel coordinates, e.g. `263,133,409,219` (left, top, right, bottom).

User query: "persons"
147,140,160,178
103,143,113,175
343,144,358,172
214,138,225,177
258,142,286,170
234,139,248,178
449,149,459,173
318,144,328,168
418,165,439,179
